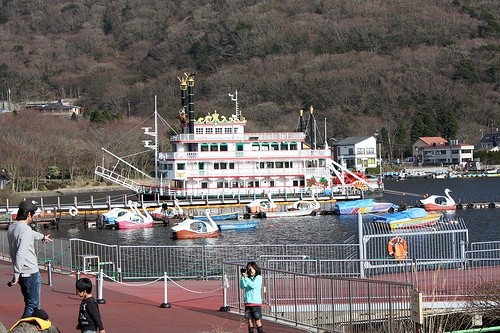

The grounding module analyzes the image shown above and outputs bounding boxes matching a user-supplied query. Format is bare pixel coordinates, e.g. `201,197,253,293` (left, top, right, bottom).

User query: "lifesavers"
69,207,78,217
388,237,408,257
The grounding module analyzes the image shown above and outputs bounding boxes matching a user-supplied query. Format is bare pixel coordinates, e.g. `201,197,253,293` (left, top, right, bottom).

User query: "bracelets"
42,235,45,243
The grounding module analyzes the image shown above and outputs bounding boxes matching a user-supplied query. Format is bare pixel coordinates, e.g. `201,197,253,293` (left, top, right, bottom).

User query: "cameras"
240,268,250,274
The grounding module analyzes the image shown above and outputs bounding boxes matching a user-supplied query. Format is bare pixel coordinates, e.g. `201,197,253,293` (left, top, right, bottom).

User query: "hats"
19,201,38,211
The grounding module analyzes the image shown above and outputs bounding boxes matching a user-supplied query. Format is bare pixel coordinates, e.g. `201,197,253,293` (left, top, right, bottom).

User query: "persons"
75,278,105,333
7,201,53,319
162,200,168,214
239,262,263,333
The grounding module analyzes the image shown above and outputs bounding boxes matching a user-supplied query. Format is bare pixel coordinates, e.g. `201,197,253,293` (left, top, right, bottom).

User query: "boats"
372,207,444,234
285,191,324,217
92,88,500,233
333,197,400,215
245,192,279,215
171,208,220,240
188,211,239,221
419,187,456,211
216,218,260,232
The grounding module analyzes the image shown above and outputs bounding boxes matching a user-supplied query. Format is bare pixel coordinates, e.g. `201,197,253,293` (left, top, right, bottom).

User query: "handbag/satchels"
32,307,49,320
